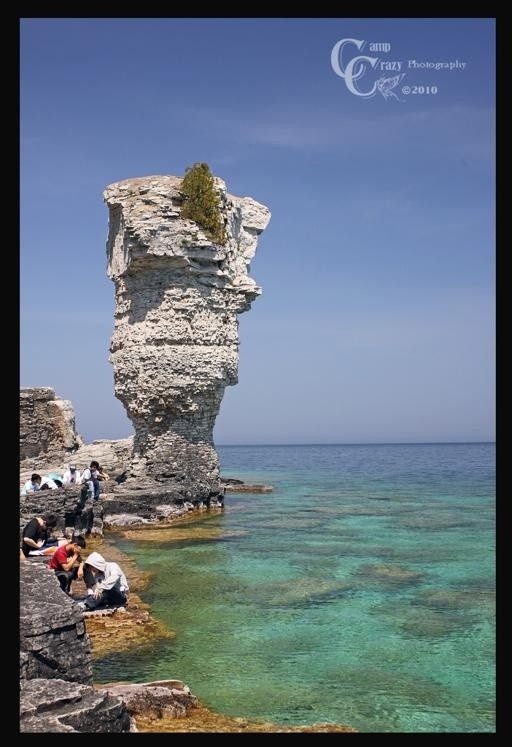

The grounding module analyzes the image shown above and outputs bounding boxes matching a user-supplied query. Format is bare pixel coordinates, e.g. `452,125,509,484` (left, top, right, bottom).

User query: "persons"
79,553,129,609
22,514,59,557
48,536,86,595
20,461,109,501
